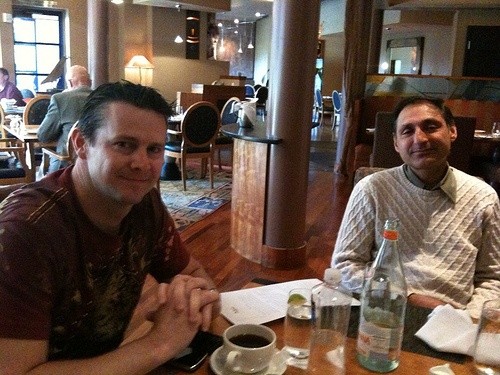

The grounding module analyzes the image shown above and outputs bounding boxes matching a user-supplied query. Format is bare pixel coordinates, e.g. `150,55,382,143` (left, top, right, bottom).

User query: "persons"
0,79,222,375
0,68,25,137
331,96,500,364
37,65,94,174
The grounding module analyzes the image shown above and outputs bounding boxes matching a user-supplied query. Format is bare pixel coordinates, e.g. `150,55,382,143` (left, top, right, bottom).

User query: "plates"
210,345,287,375
28,130,38,133
474,130,486,134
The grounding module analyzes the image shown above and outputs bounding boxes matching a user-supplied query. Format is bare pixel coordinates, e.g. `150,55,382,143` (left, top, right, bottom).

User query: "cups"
7,102,15,111
492,123,500,138
223,323,276,373
472,300,500,375
285,288,312,359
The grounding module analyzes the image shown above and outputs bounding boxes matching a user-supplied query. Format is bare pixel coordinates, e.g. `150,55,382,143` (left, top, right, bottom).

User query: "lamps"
125,56,154,86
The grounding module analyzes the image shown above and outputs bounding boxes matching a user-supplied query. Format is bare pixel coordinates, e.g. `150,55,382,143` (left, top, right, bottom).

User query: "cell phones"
170,329,224,371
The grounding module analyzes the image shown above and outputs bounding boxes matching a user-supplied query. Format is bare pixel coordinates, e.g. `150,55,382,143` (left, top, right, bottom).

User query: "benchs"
349,99,500,171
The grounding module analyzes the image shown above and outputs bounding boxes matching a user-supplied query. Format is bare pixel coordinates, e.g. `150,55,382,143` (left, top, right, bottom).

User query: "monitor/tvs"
462,25,500,80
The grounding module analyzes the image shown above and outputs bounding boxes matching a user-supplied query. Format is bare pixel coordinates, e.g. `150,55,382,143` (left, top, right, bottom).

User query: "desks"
148,279,500,375
3,125,50,178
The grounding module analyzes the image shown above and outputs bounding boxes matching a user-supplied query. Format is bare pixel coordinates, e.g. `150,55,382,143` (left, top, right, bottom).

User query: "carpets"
159,164,232,230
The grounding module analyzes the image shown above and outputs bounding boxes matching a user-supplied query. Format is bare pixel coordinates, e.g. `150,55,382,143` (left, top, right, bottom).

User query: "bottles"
237,101,256,127
356,220,407,372
310,268,352,375
9,118,21,128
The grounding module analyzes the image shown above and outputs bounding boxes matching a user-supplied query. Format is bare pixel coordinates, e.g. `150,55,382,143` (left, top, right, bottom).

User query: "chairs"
245,83,269,122
163,100,221,191
313,89,334,129
0,95,80,183
331,89,342,130
